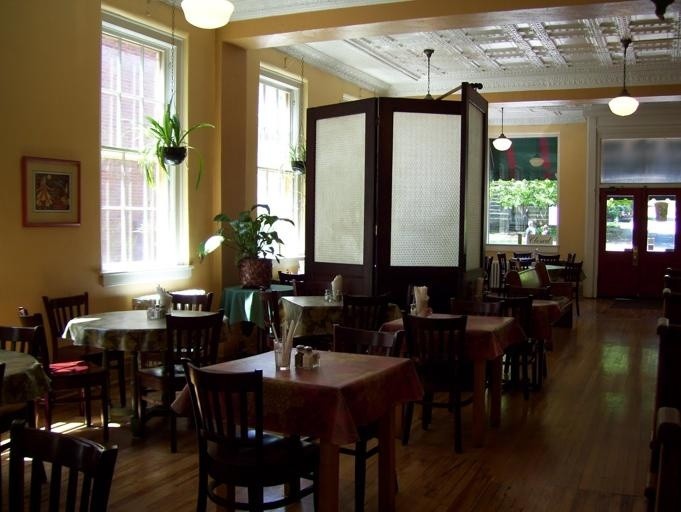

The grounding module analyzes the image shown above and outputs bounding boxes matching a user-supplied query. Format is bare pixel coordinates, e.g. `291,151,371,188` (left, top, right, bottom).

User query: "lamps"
489,103,516,154
604,36,641,118
178,0,236,32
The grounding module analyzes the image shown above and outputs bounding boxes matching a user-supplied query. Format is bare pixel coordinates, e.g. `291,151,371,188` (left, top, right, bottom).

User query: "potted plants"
287,140,307,176
208,199,296,290
137,90,218,194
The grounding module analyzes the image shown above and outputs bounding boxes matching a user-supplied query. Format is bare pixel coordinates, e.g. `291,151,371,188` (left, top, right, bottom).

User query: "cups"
415,297,430,316
274,338,290,371
157,296,172,312
332,281,343,299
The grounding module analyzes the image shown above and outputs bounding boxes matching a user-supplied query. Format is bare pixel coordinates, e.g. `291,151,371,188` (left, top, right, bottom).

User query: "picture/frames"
18,152,83,230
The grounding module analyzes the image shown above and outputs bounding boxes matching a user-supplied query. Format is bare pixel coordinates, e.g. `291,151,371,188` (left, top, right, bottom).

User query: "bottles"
410,303,416,315
325,289,343,303
147,304,166,321
295,344,304,367
304,346,313,368
